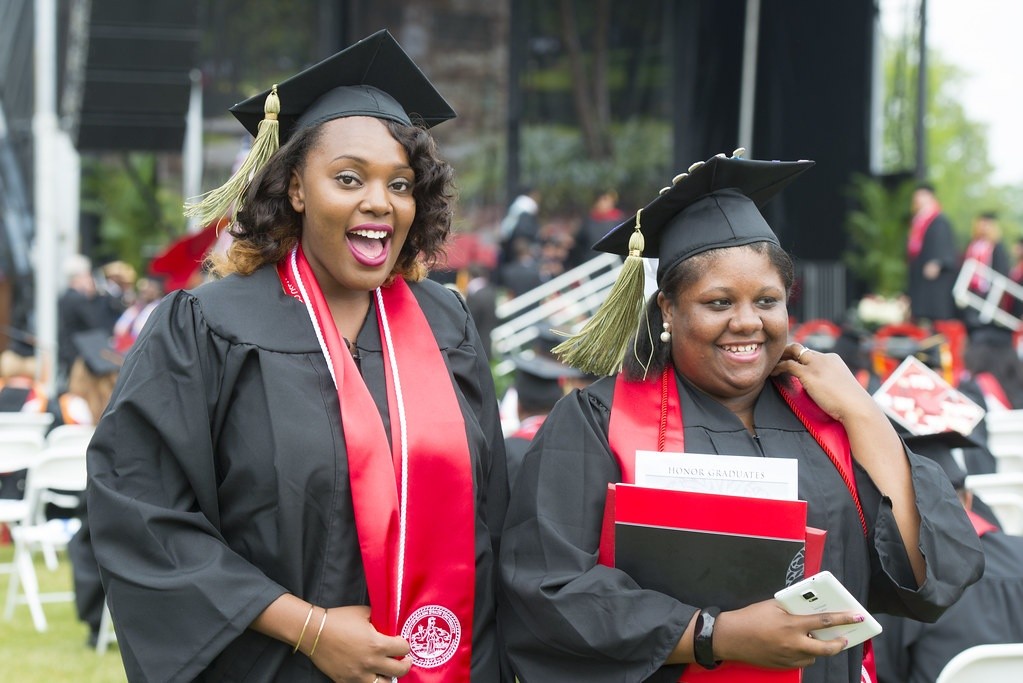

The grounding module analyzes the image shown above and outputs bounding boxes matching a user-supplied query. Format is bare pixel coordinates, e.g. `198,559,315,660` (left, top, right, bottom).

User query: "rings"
373,674,379,683
797,346,810,360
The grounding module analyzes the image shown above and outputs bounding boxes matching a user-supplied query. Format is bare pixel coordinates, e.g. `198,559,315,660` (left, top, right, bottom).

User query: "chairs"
0,410,118,652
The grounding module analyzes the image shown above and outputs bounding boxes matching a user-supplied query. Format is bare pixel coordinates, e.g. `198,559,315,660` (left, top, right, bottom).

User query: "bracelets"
308,607,328,658
693,606,724,670
292,604,315,655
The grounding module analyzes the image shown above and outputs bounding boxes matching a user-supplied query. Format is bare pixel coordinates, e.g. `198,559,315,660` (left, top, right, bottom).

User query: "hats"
550,148,816,380
514,357,585,411
182,29,457,237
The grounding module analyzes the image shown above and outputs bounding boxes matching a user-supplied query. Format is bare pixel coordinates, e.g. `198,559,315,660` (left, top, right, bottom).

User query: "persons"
906,183,957,327
57,249,163,366
900,429,1023,683
455,263,495,364
495,148,985,683
563,182,633,281
497,180,575,301
954,327,1023,413
504,355,588,496
85,26,518,683
963,207,1015,348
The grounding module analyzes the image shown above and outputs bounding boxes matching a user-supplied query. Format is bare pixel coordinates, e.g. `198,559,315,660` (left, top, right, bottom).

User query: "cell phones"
774,570,882,650
